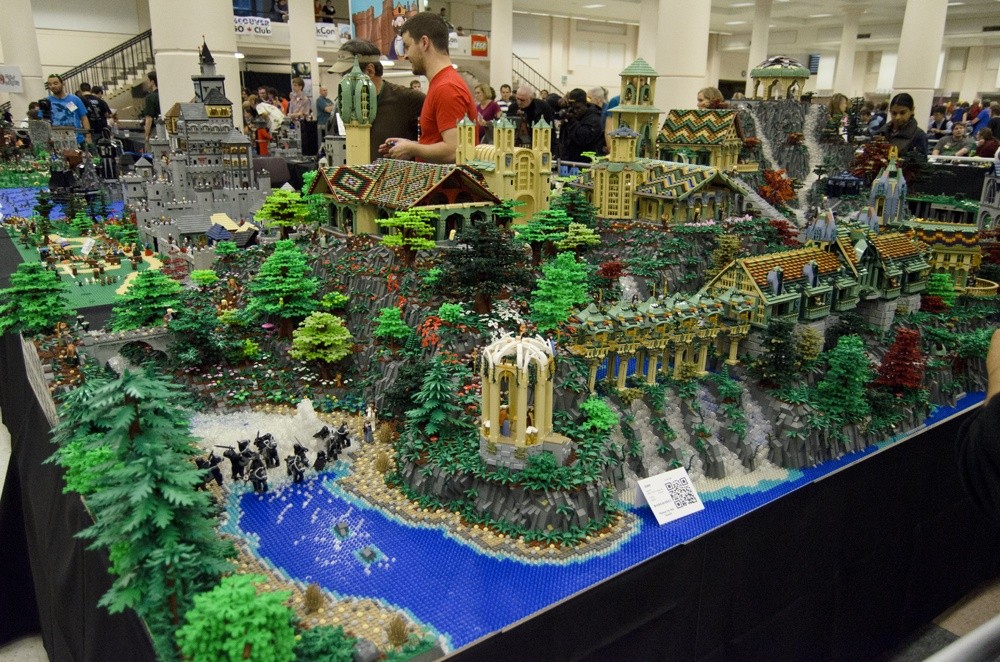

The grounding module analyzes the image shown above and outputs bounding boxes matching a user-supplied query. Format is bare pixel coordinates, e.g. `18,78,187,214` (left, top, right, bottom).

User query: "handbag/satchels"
102,127,111,139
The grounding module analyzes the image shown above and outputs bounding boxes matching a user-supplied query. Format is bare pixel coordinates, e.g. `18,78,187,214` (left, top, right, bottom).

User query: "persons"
317,86,334,147
410,81,421,90
438,8,448,26
875,93,928,162
20,101,39,129
75,83,112,144
826,93,848,142
927,99,1000,158
37,74,93,150
314,0,322,22
241,86,289,140
860,103,888,136
287,77,310,122
697,87,724,109
473,84,620,172
271,0,288,22
144,71,160,152
328,39,427,163
378,11,479,165
322,0,336,23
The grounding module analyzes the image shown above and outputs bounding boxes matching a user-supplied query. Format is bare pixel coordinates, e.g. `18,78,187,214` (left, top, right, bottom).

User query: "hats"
327,50,380,74
568,89,587,103
